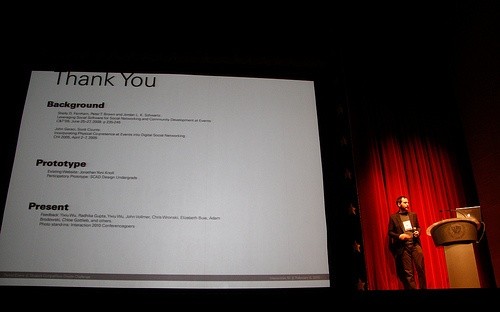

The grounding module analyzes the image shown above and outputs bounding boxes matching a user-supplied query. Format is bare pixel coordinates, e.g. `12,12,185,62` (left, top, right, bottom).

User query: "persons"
387,195,428,290
345,203,369,291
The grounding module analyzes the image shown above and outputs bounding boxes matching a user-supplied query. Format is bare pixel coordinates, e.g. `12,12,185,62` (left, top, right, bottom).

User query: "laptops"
456,206,482,223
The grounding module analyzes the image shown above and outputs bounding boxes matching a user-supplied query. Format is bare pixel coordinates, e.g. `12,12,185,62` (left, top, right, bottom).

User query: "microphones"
448,209,468,219
